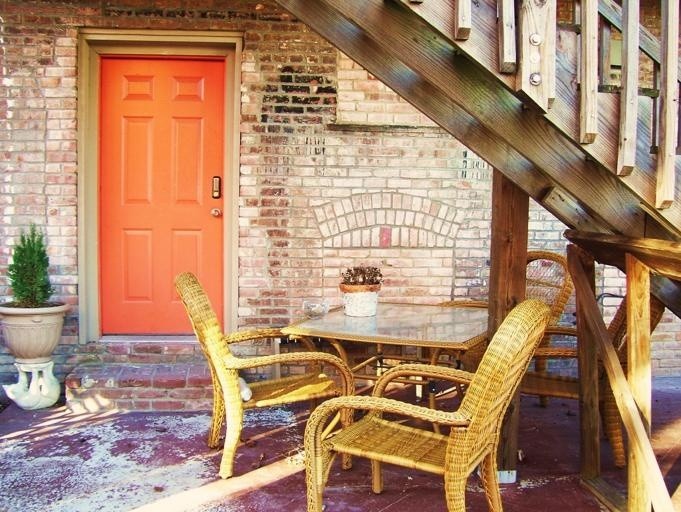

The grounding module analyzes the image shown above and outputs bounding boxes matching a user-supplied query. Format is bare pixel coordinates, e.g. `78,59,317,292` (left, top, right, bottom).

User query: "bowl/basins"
302,299,330,319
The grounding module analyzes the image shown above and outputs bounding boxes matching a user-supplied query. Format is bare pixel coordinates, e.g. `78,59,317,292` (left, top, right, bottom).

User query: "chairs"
400,252,664,471
305,297,552,512
174,270,372,479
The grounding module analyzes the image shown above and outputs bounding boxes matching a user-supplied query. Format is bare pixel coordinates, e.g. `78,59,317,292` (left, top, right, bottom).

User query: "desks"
280,302,488,433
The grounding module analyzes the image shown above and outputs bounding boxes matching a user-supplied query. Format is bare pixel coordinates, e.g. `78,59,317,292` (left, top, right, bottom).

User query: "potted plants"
0,222,70,363
